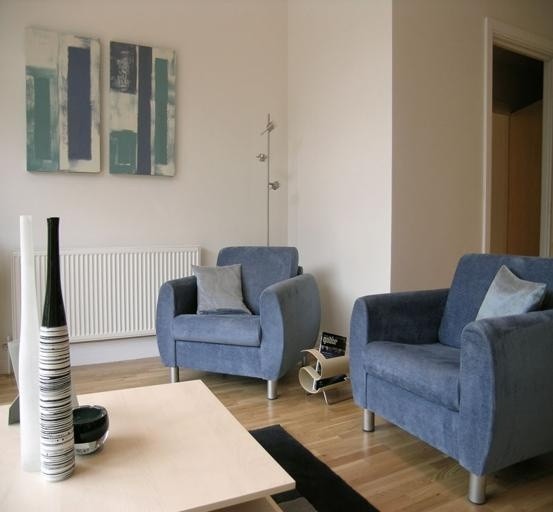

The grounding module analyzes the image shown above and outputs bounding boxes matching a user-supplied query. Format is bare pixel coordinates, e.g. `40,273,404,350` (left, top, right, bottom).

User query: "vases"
15,214,45,477
37,215,80,485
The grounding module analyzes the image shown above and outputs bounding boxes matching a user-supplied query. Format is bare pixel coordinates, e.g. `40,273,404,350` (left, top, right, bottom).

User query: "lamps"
255,112,280,246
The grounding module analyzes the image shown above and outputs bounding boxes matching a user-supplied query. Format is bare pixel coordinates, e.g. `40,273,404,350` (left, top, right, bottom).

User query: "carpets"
246,424,378,512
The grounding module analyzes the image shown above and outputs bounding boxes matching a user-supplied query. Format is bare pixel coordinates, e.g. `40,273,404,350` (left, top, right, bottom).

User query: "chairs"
157,245,318,399
347,251,550,505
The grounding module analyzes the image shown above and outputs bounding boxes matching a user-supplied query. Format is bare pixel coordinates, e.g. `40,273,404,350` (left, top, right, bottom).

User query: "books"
315,332,347,373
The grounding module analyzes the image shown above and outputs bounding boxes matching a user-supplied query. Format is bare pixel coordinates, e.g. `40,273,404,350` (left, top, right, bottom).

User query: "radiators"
11,247,202,345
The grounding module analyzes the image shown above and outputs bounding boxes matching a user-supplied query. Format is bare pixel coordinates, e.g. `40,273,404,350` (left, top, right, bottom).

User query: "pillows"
189,261,253,315
474,264,549,322
